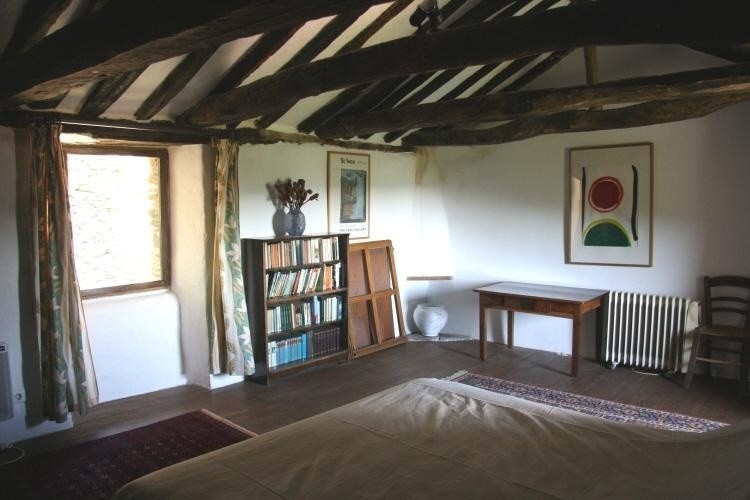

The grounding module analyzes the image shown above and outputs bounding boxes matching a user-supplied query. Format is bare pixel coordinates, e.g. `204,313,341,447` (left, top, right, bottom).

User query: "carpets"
1,407,259,499
440,370,731,432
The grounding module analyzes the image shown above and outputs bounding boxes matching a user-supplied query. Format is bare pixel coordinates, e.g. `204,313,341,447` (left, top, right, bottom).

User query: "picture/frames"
326,150,370,239
568,144,652,268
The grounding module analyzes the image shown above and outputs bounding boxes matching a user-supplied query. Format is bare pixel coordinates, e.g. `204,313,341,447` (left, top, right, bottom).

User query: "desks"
472,280,610,376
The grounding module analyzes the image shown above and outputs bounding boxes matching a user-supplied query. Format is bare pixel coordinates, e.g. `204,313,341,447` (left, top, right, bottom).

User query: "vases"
412,300,448,338
285,210,306,236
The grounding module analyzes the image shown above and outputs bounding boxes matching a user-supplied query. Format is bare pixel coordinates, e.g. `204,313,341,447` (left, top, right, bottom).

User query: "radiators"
604,291,692,377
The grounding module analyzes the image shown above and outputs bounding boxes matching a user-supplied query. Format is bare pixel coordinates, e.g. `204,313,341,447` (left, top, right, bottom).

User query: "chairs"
684,276,749,396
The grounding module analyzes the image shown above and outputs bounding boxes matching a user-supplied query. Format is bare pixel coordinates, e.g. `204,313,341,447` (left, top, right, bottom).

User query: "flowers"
273,178,319,209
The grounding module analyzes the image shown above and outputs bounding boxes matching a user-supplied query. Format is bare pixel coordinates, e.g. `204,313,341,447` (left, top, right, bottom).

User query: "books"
264,236,343,368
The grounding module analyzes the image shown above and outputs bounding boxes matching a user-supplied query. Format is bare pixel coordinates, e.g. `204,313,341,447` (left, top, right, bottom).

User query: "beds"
110,377,749,498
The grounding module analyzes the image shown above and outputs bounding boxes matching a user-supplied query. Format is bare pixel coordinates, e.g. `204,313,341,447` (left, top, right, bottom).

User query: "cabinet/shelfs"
240,234,349,385
